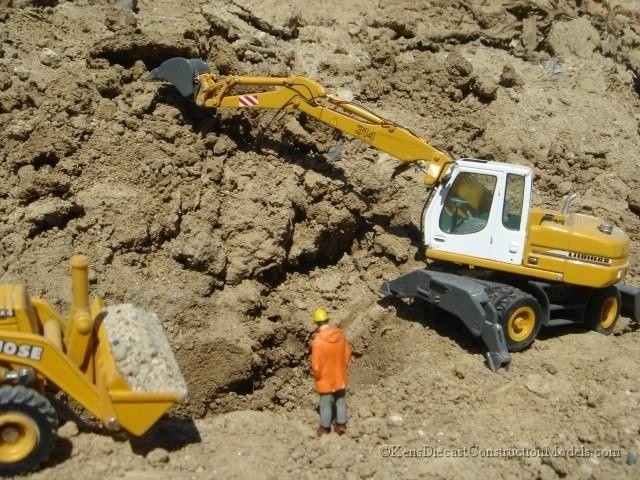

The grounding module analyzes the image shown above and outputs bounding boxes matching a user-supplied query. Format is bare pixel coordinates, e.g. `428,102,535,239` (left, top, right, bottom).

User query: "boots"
316,421,346,437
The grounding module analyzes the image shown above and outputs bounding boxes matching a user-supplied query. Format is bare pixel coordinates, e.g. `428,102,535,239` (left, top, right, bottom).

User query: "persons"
152,56,640,374
311,309,352,436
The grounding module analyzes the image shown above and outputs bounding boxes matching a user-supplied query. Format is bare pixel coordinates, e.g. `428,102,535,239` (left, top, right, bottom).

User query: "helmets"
312,309,330,322
